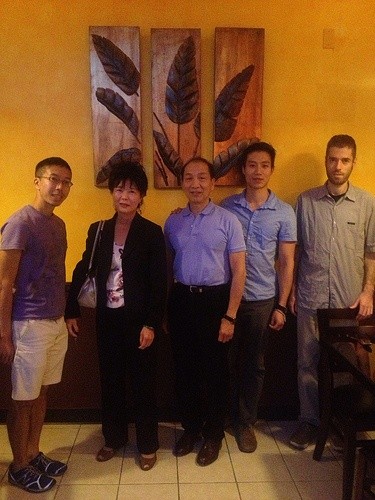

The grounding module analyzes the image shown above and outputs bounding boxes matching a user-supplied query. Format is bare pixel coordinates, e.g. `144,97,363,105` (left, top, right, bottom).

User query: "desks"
320,338,375,392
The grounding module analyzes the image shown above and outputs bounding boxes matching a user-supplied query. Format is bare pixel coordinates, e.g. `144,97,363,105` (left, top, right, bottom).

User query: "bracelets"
143,324,155,331
277,309,286,325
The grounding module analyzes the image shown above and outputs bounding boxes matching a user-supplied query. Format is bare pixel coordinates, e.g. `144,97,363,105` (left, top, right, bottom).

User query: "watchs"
275,304,289,316
224,314,238,325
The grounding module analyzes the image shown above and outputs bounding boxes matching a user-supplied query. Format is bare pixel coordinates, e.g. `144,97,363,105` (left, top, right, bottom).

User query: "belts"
176,281,226,293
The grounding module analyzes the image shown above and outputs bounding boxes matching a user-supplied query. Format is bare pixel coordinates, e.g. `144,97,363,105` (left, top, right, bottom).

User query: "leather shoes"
197,437,222,466
172,427,203,457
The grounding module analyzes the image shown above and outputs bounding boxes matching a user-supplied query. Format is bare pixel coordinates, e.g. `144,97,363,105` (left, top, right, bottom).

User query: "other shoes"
289,421,316,448
234,421,257,453
96,440,125,462
137,451,157,471
329,429,346,451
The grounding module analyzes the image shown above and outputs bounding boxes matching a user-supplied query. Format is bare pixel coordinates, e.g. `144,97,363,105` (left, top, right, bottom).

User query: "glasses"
38,176,73,187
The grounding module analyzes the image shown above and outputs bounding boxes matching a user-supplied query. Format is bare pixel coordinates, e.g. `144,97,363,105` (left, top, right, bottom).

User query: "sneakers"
29,452,67,476
8,462,56,493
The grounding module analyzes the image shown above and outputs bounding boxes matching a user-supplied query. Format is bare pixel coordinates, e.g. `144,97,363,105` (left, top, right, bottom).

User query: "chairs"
313,305,375,500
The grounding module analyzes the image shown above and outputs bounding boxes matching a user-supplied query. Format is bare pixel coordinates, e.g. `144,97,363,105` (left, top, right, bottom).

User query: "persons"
68,162,167,471
159,157,246,468
290,134,375,450
0,157,73,492
168,141,298,452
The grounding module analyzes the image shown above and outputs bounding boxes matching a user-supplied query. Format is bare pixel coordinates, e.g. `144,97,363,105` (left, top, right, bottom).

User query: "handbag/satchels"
76,219,105,308
